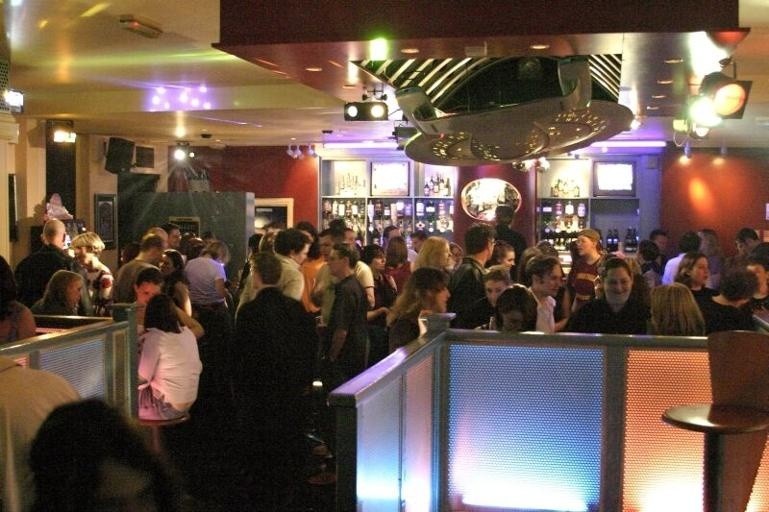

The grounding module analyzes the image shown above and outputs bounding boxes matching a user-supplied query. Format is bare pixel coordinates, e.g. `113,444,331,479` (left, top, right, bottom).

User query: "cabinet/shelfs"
317,142,459,259
536,139,667,274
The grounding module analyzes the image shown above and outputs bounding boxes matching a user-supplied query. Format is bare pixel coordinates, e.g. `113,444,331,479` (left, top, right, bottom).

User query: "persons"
0,214,768,460
493,206,528,281
27,397,193,512
0,353,81,512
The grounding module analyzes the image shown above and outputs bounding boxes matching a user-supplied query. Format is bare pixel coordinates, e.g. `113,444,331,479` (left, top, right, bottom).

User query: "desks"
662,404,769,512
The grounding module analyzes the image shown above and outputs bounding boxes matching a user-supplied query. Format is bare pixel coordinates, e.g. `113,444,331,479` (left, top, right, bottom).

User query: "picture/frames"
254,198,294,236
95,193,117,250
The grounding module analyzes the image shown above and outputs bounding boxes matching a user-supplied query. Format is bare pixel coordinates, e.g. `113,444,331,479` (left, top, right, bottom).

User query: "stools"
134,412,191,457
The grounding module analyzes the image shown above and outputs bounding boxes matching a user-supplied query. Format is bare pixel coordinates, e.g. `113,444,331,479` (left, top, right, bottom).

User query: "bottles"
335,172,366,196
424,172,450,197
542,202,587,253
597,227,638,253
324,199,455,247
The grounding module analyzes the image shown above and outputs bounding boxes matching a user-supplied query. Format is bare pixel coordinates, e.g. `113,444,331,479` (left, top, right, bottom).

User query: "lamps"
118,14,163,39
286,140,316,160
173,139,195,167
344,87,389,121
45,118,77,145
671,27,753,158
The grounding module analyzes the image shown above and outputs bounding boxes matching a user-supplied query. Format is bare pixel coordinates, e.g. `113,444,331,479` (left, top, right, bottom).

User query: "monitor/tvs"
593,161,637,197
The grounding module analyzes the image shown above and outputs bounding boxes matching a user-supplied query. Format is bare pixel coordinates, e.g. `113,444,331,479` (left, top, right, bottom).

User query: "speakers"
136,146,154,167
105,137,135,174
